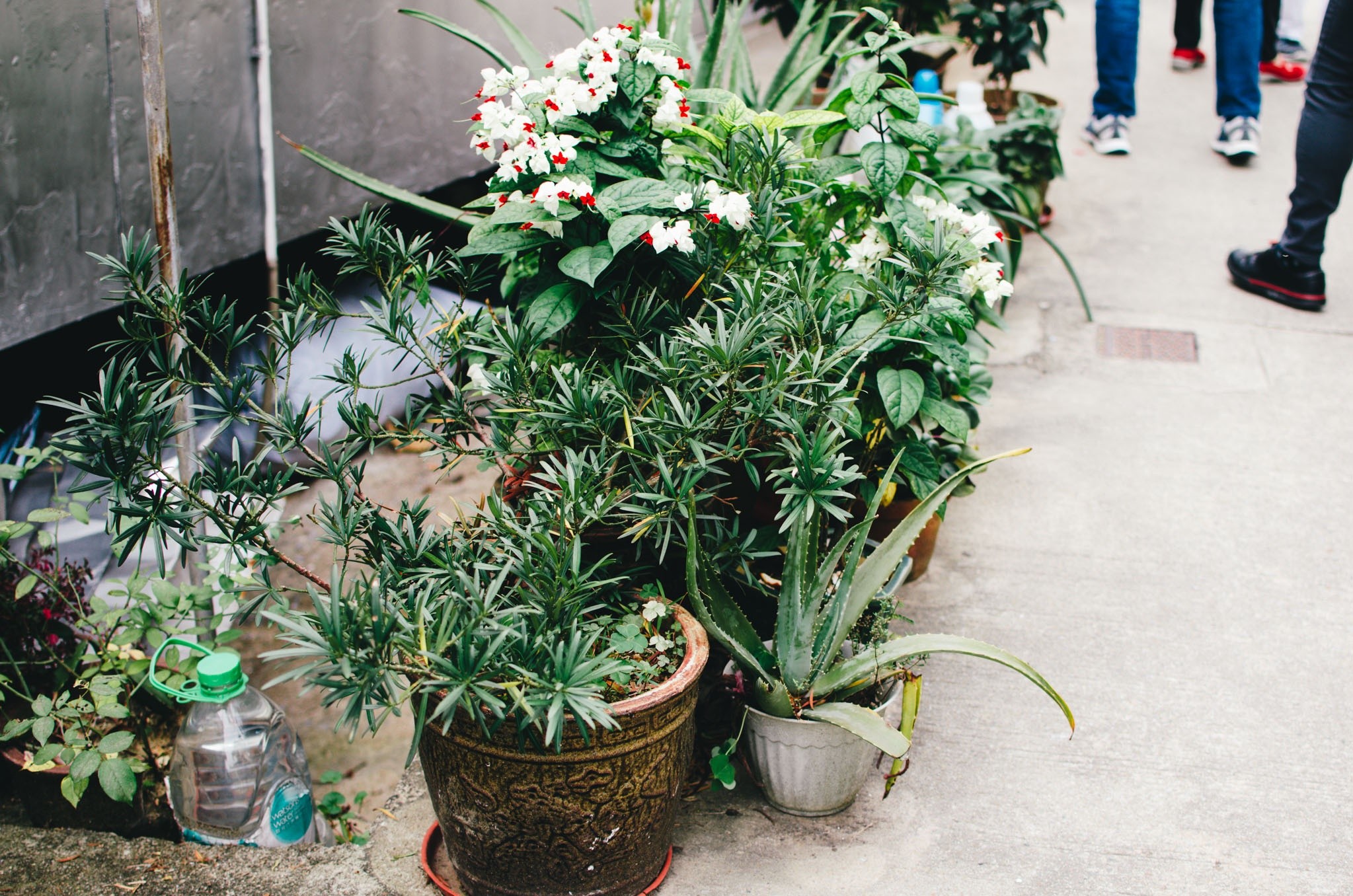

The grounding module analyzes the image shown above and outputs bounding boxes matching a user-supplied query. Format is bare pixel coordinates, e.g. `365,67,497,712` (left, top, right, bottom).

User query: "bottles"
913,70,996,130
148,638,321,845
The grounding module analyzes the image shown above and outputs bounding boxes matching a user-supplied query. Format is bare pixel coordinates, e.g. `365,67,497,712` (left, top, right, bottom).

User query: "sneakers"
1080,114,1130,154
1226,240,1326,309
1210,114,1262,157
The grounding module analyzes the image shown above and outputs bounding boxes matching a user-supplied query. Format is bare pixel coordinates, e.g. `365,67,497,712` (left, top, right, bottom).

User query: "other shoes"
1274,33,1308,61
1022,204,1055,231
1172,47,1205,70
1255,60,1307,82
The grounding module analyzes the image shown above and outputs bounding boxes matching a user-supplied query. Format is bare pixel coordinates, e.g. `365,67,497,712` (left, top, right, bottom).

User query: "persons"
1078,0,1268,161
1226,0,1353,313
1170,0,1308,88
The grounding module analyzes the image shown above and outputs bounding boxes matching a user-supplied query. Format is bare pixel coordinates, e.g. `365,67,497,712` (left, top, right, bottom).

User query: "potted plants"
30,3,1078,892
